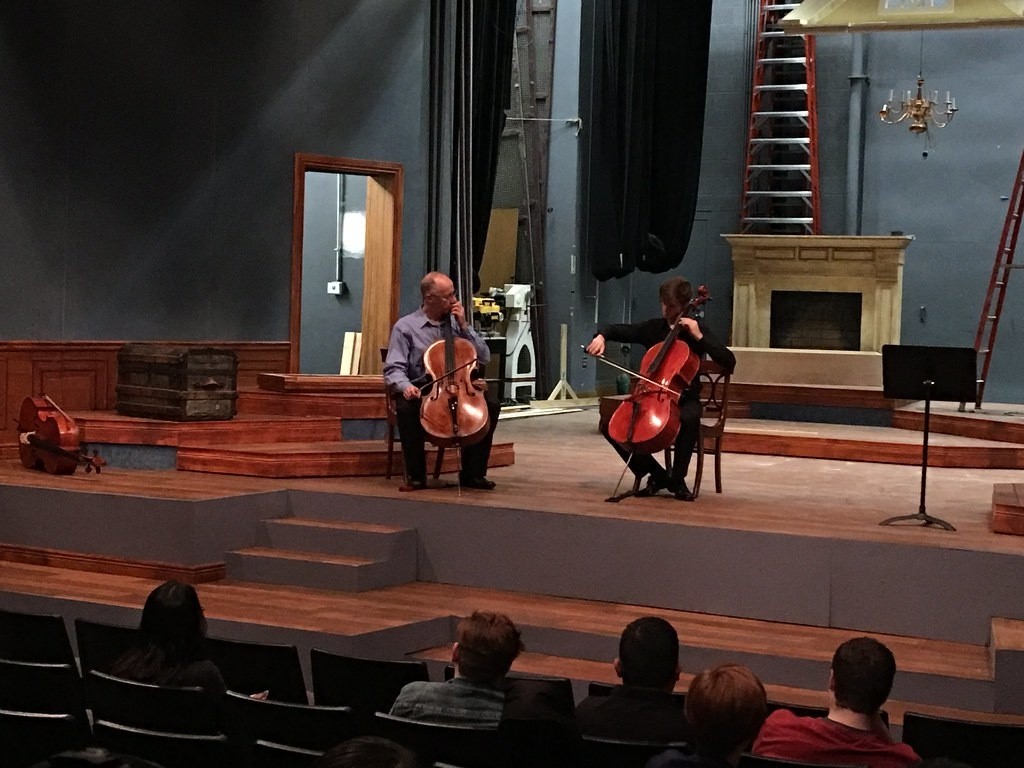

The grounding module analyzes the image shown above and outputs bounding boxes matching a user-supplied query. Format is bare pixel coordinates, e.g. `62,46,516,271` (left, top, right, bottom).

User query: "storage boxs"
116,343,239,420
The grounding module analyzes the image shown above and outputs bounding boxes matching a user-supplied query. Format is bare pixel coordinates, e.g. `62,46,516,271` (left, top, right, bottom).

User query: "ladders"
739,0,821,236
958,144,1024,412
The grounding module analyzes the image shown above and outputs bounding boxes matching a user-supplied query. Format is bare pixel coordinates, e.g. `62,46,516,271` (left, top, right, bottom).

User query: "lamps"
878,27,957,156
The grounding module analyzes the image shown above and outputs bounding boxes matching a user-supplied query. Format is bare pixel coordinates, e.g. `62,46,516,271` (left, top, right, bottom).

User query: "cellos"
16,394,108,476
416,312,491,498
606,285,714,498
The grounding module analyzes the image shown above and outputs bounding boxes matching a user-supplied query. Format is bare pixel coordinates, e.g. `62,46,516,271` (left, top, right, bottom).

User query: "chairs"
609,348,735,504
0,607,1024,768
380,336,487,479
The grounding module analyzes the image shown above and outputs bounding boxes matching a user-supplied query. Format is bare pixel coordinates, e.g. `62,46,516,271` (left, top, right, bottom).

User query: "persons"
586,277,736,501
644,662,769,768
748,637,923,768
109,579,225,693
318,736,417,768
390,612,553,728
383,272,496,491
577,617,696,743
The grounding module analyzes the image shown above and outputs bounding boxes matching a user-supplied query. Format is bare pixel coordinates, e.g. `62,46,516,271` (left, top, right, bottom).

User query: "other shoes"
637,471,669,497
399,479,426,492
668,476,693,501
460,475,496,488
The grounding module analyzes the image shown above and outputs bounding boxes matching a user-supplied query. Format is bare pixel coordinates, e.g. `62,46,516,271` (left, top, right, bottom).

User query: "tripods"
882,344,977,532
